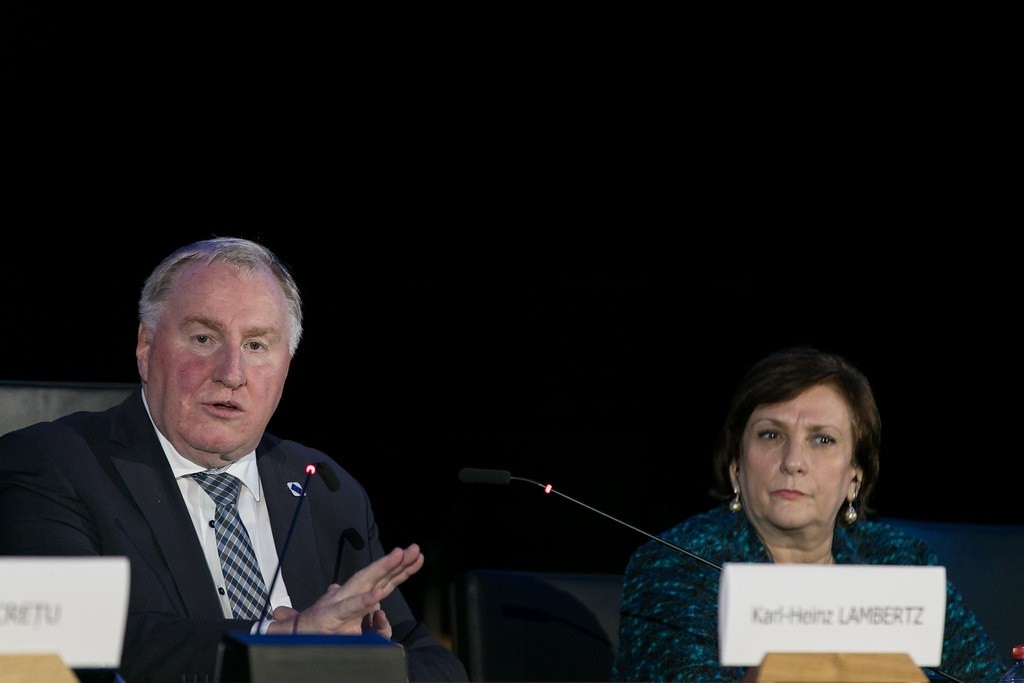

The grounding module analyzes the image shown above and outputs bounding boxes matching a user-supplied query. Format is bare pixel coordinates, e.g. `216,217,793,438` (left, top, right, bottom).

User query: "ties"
191,472,273,621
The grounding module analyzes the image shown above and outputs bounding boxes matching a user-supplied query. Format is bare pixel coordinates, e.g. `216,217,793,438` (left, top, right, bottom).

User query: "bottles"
997,644,1024,683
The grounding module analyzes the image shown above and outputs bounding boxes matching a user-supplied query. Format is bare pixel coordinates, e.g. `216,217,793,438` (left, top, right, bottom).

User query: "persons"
0,235,467,683
613,345,1024,683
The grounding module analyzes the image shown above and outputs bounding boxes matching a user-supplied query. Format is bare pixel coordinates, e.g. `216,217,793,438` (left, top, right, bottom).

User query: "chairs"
464,570,623,683
888,519,1023,668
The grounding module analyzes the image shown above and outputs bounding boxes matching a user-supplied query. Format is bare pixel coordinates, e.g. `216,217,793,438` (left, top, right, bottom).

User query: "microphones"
459,468,724,573
254,460,340,635
